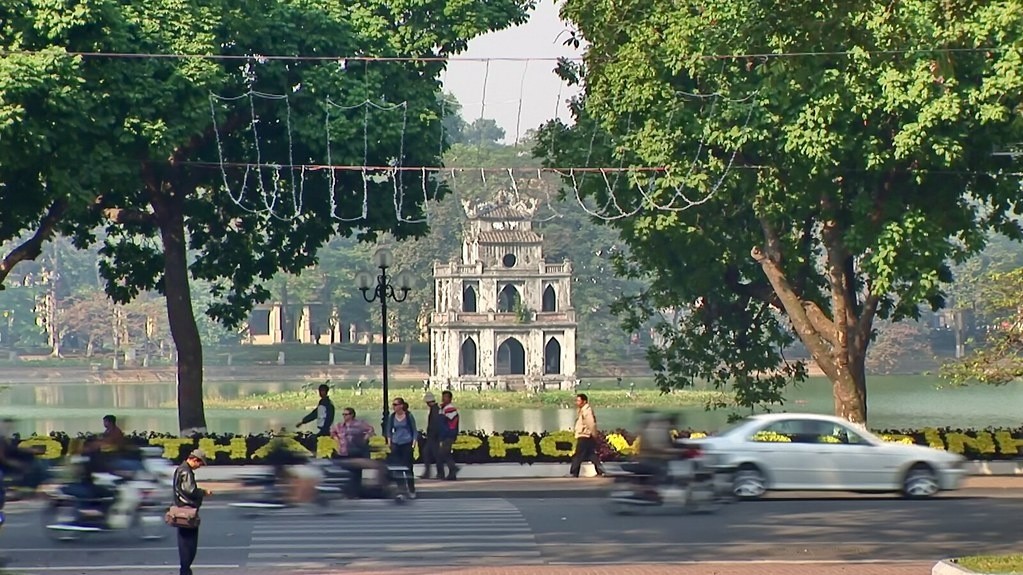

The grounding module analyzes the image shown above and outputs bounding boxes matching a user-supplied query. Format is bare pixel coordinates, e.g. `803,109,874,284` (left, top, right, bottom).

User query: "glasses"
343,414,351,416
392,403,401,406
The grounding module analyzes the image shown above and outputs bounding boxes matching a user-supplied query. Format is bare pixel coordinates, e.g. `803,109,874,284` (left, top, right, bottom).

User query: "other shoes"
423,474,432,476
397,493,408,499
596,474,602,477
563,474,578,478
436,473,441,476
447,467,460,478
442,475,456,481
409,491,416,498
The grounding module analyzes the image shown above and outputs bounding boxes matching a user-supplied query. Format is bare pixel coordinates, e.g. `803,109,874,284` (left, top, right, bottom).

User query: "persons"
292,385,460,503
88,414,126,449
173,449,214,575
569,392,609,479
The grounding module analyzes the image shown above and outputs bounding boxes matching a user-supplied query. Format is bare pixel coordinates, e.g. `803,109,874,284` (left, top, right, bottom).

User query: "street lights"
354,248,417,444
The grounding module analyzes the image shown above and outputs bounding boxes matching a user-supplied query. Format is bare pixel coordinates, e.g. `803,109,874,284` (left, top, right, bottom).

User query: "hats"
424,392,435,402
191,449,207,466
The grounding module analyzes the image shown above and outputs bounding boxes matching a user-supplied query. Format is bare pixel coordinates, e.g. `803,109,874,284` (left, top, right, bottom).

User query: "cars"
673,412,968,500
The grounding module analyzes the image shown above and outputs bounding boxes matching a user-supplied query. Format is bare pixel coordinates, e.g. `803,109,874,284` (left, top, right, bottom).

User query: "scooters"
40,446,180,545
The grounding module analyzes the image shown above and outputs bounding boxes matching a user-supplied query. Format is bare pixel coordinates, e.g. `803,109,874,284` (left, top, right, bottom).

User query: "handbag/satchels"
165,506,201,528
348,437,370,458
